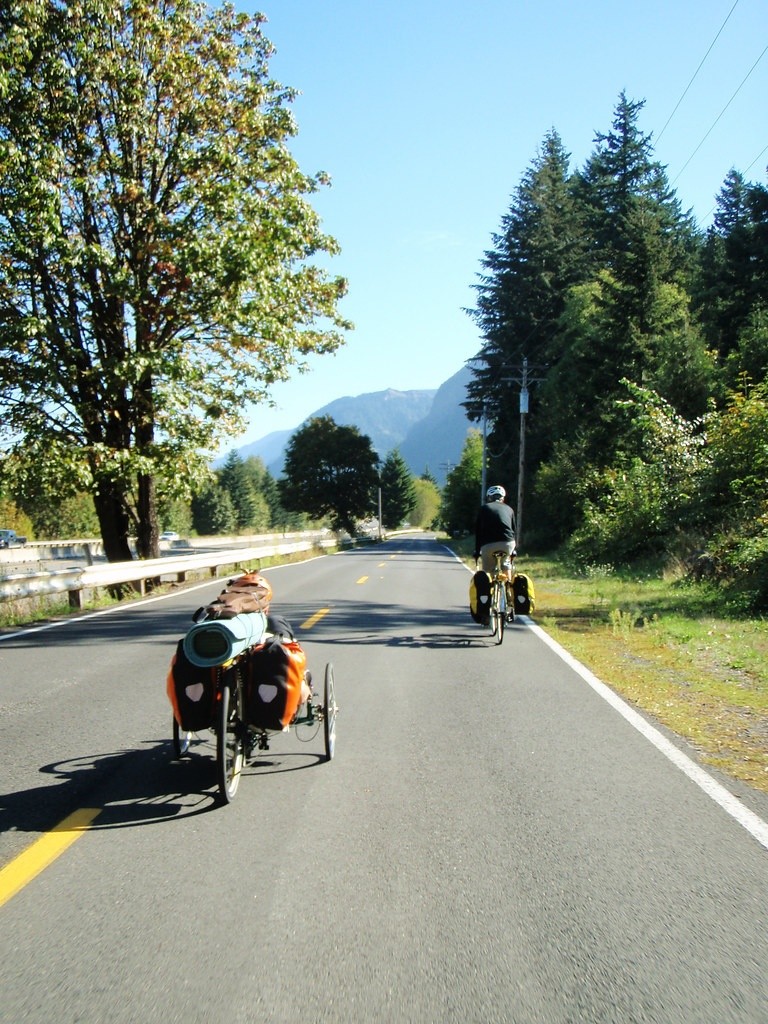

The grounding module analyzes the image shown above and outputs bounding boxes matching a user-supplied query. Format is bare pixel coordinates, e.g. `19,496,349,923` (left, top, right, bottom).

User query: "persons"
474,486,517,580
233,569,312,705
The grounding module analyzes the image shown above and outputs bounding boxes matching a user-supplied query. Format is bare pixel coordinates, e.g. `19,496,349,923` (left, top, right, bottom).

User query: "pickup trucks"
0,529,27,549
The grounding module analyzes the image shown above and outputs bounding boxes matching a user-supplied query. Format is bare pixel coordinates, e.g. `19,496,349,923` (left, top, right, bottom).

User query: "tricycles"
174,579,340,804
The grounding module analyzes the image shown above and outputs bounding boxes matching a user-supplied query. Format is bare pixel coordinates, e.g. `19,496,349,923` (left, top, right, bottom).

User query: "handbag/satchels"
247,635,306,731
468,571,492,625
168,638,220,730
511,572,535,615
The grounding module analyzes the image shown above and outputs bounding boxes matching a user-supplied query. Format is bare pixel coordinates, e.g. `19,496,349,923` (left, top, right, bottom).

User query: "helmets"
487,485,507,497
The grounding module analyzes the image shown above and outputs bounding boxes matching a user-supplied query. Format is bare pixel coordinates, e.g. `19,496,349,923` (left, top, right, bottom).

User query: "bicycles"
473,547,521,646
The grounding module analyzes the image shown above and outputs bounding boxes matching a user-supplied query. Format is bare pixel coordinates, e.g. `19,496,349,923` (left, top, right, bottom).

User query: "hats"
232,574,273,601
